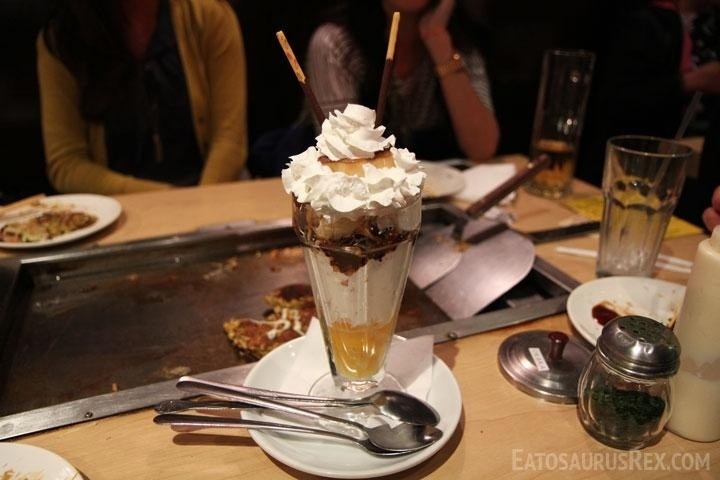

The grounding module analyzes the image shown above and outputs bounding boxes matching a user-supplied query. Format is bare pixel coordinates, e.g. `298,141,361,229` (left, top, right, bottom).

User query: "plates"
567,275,687,349
0,193,122,248
239,333,463,478
0,441,86,480
419,161,467,199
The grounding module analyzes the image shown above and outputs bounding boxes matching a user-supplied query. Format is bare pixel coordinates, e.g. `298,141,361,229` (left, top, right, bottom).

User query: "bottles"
663,224,719,442
575,315,681,448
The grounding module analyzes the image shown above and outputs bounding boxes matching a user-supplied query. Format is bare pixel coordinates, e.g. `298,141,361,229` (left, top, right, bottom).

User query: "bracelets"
434,52,471,78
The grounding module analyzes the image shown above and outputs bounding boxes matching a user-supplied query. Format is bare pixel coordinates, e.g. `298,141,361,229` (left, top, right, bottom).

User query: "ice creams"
282,101,427,378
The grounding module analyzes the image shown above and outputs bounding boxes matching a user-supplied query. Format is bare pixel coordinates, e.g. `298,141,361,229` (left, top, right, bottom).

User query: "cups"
594,135,694,280
521,50,596,202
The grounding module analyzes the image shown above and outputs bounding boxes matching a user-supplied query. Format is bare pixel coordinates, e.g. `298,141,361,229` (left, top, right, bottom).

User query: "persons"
289,0,503,163
587,0,720,233
36,1,252,195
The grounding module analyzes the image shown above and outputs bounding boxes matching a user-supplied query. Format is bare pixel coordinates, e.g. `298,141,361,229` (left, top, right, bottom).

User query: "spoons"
178,374,442,425
152,413,437,457
154,389,441,426
176,380,443,451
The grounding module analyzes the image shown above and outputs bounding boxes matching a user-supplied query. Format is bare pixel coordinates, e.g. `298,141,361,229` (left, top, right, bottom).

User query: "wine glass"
289,180,421,414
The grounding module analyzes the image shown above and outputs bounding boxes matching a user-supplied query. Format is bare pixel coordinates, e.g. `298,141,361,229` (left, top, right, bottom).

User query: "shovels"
407,152,552,292
422,215,601,321
